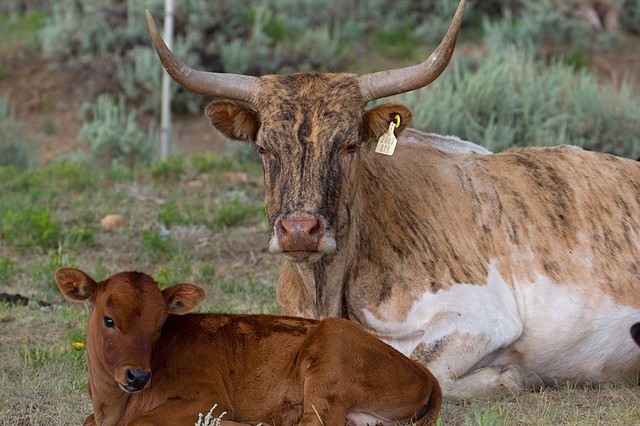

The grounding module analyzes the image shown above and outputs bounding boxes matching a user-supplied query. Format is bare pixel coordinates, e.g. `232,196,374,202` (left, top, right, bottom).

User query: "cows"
54,269,442,426
145,0,640,401
